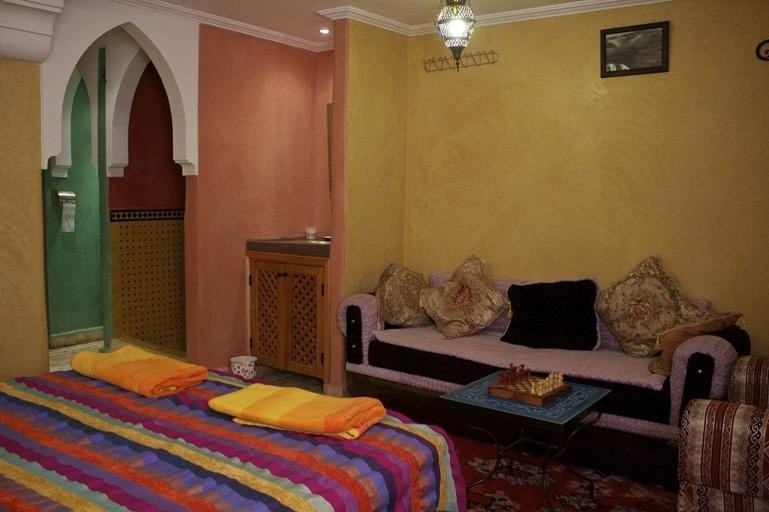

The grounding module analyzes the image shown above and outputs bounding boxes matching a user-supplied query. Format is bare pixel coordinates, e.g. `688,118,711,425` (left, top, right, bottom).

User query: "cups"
306,224,317,240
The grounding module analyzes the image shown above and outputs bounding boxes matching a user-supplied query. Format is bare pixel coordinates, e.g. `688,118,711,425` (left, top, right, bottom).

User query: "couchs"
674,358,769,512
339,273,749,446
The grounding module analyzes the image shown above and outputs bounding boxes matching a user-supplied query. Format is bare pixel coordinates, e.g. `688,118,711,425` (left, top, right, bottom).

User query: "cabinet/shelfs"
246,250,329,380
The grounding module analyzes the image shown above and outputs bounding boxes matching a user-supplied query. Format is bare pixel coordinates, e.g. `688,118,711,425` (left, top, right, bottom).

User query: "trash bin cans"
230,355,258,381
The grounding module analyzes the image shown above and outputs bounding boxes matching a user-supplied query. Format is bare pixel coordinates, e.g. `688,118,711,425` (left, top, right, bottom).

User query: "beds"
2,365,470,512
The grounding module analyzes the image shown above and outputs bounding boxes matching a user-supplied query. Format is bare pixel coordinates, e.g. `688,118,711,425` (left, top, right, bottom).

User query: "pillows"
376,263,432,326
500,278,600,351
651,312,742,374
421,256,510,339
594,257,711,359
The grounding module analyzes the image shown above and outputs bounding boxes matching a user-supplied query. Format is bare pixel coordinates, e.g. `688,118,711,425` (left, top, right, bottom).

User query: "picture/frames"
600,20,670,78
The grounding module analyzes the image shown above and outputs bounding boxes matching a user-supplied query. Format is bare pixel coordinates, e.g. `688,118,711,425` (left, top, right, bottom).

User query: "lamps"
432,2,475,72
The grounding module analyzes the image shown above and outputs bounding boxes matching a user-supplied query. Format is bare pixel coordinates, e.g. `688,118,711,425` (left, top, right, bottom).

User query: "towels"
66,344,210,401
208,384,386,440
